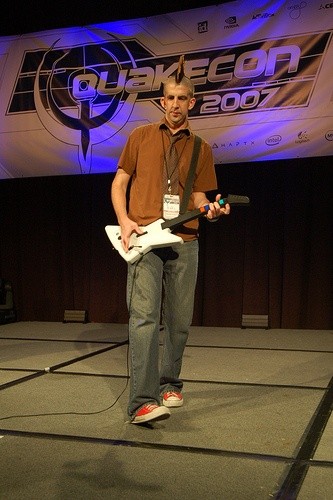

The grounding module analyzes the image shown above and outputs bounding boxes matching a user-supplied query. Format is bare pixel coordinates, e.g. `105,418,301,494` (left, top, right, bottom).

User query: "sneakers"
162,388,184,406
130,403,171,423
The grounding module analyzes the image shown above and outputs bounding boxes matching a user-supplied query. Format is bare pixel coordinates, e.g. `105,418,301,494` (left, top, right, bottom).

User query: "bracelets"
206,215,220,222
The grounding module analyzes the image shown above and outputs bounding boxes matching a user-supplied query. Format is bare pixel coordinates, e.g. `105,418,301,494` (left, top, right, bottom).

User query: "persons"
112,55,230,424
